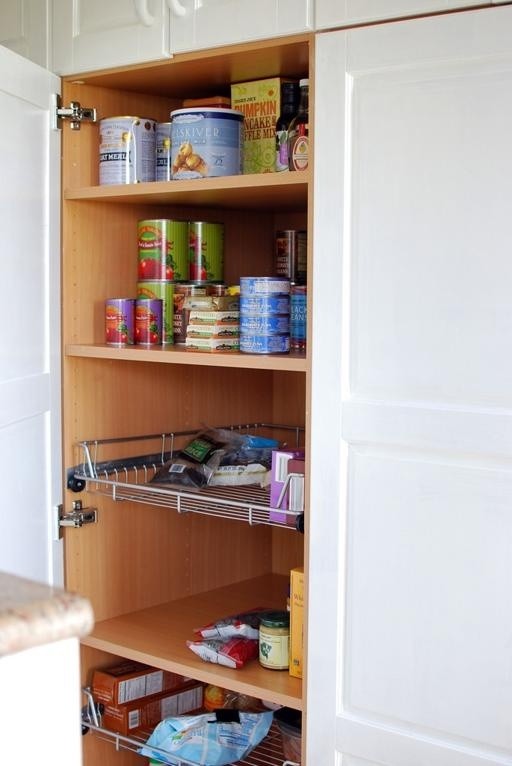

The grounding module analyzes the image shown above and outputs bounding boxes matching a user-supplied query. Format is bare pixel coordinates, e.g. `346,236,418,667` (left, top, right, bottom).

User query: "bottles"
289,285,307,352
137,219,189,283
259,610,290,671
134,298,162,347
174,283,212,346
97,115,157,187
212,284,241,297
272,228,308,286
187,221,224,283
138,284,175,344
156,123,173,182
275,80,297,174
231,79,291,175
288,76,309,173
105,298,134,345
169,108,244,179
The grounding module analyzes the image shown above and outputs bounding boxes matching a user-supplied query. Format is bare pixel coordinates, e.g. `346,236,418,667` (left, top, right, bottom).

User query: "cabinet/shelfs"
54,1,314,75
0,2,508,765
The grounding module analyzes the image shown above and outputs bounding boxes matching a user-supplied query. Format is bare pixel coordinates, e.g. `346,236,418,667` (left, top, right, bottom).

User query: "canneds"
185,340,241,353
188,312,240,326
240,276,290,355
187,326,240,339
183,296,240,312
